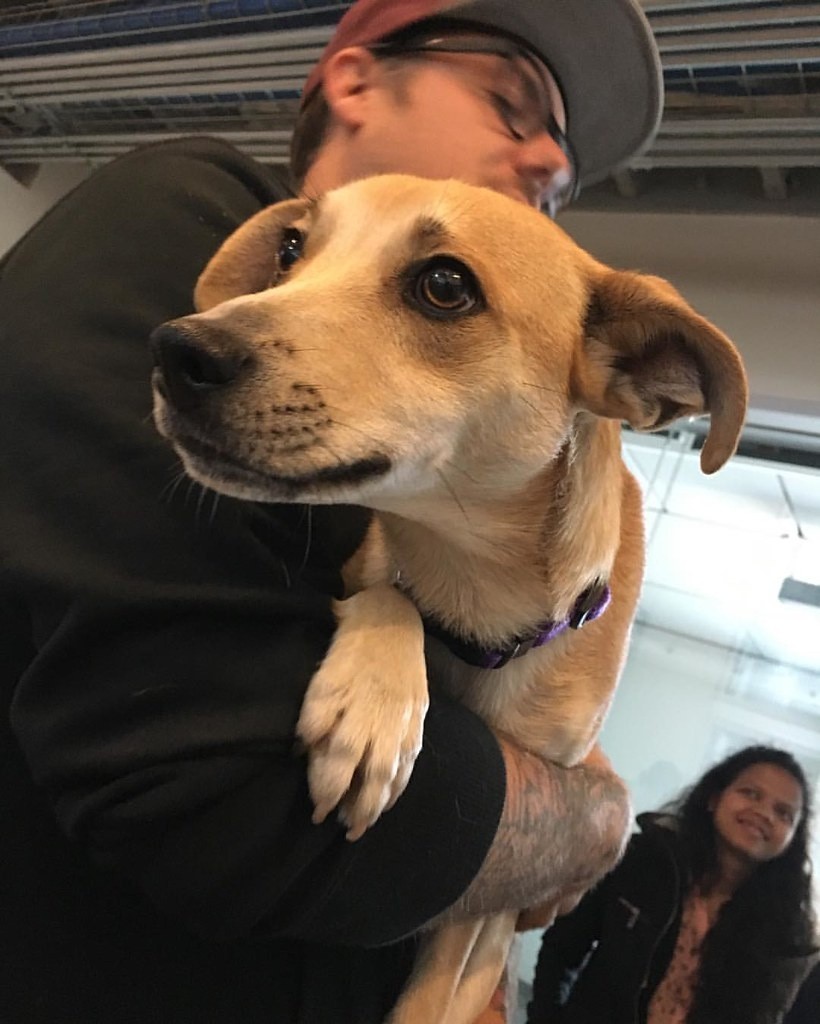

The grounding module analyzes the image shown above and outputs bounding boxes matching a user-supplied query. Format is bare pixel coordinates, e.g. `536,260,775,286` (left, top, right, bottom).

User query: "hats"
297,0,665,188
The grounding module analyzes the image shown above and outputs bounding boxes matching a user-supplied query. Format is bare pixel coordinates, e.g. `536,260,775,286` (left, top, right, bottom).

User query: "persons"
527,746,820,1024
0,0,663,1024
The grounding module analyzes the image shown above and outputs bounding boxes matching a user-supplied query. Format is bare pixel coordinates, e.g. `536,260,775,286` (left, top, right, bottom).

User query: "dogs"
147,172,749,1024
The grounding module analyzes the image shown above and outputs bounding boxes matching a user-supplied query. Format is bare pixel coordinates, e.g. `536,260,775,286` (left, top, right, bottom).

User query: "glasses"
367,44,581,221
